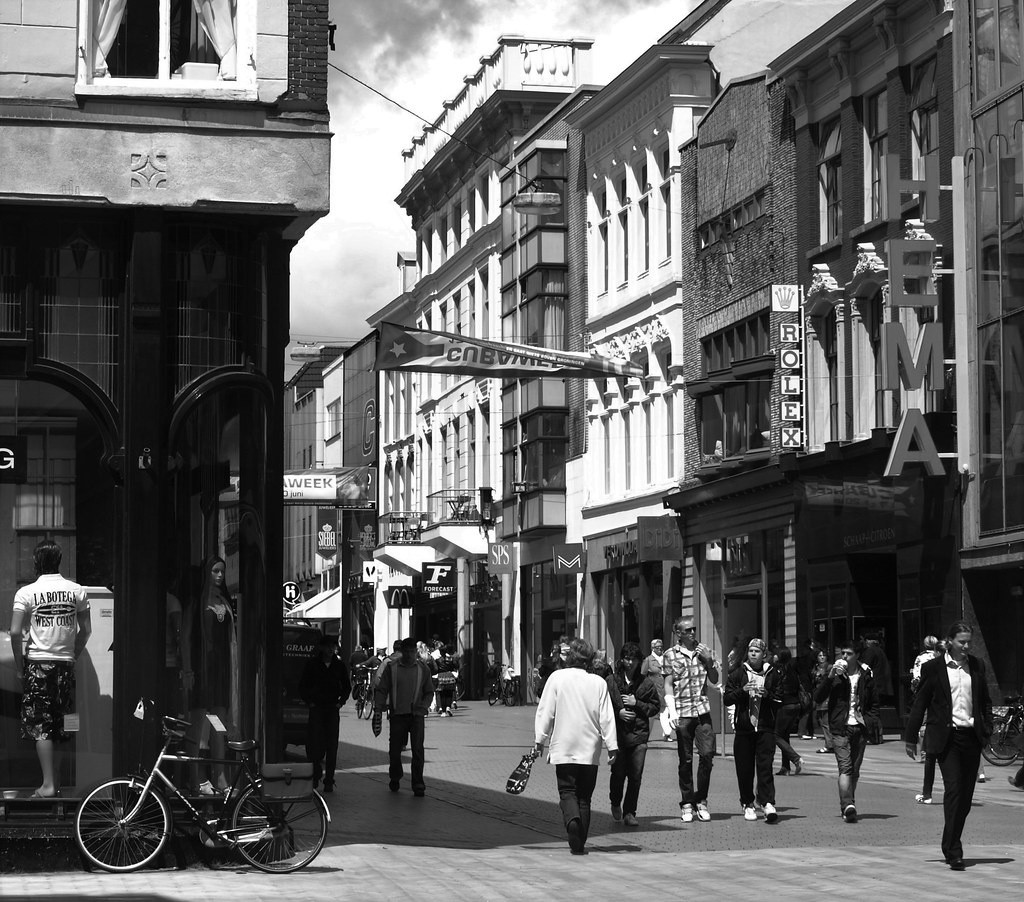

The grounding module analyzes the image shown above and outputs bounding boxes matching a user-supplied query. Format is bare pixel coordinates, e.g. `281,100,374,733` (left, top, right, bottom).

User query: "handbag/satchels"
506,755,534,794
372,712,383,738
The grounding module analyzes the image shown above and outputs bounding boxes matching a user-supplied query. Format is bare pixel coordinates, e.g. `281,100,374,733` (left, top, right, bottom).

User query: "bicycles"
353,664,380,720
980,695,1024,766
453,676,466,701
74,716,330,875
488,662,520,707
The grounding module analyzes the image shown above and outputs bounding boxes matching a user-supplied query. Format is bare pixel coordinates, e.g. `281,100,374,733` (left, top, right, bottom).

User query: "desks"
444,500,466,521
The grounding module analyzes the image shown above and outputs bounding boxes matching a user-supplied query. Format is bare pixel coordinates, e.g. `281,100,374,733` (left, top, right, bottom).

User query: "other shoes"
816,747,833,753
568,820,584,855
424,700,457,717
844,804,857,822
324,782,334,792
389,777,400,792
977,774,986,783
744,807,758,820
762,803,777,823
948,857,965,870
915,793,933,803
1008,776,1024,791
414,788,425,798
313,778,319,788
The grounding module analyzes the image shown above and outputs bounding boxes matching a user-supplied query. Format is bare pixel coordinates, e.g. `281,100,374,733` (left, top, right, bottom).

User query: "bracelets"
182,669,194,677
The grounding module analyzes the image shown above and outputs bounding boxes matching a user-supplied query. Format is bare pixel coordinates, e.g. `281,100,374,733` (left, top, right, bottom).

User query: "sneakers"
680,804,693,823
611,805,622,820
624,813,639,825
696,803,711,821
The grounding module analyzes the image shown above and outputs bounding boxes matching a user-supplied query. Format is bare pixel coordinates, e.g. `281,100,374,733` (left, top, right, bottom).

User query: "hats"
401,638,417,648
748,638,768,653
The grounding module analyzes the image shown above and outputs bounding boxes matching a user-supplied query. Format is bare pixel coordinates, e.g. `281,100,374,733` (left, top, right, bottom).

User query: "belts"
954,727,966,731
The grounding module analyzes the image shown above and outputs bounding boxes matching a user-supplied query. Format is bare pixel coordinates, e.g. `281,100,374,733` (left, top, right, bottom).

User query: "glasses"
685,627,696,633
652,646,664,649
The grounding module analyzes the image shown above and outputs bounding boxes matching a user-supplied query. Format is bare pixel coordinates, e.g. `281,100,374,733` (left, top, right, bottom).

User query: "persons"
11,540,91,799
662,617,718,822
533,639,620,855
813,641,879,824
349,634,1024,805
724,638,784,822
181,556,237,796
166,575,182,713
606,642,659,827
905,622,993,869
300,634,352,791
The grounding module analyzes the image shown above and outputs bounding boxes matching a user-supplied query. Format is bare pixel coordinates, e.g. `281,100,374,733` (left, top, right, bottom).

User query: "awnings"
287,586,342,619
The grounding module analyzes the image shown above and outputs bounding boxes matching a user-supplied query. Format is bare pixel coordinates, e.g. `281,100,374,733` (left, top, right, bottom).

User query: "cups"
836,659,848,675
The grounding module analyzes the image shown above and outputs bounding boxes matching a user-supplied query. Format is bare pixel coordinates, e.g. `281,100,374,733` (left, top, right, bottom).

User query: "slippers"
30,787,55,798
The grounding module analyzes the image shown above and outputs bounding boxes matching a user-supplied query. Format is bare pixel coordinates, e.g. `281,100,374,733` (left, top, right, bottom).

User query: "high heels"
775,766,792,776
795,763,802,775
